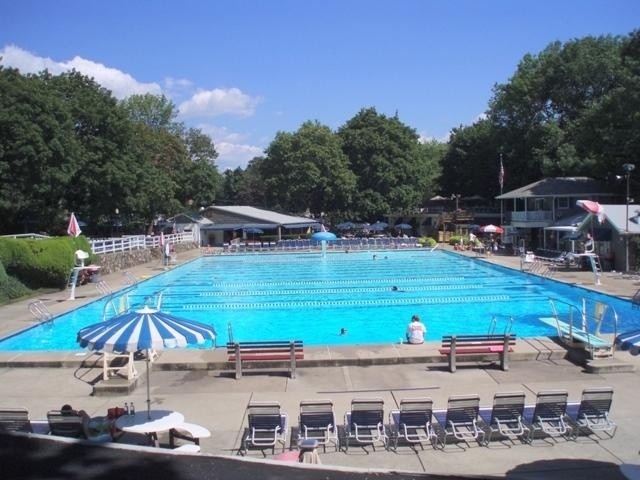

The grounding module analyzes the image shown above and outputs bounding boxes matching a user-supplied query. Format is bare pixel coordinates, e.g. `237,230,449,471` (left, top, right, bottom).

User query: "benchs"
227,340,304,379
438,333,516,373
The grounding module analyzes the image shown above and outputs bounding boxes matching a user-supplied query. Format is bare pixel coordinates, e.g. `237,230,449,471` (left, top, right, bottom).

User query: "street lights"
622,163,635,272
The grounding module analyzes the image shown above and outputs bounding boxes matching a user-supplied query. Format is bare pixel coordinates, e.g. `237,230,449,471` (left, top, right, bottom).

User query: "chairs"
297,398,340,453
226,236,422,253
344,398,388,453
555,387,617,440
427,394,485,450
534,247,566,263
388,397,437,452
0,407,115,442
472,391,529,446
244,402,287,455
511,390,572,446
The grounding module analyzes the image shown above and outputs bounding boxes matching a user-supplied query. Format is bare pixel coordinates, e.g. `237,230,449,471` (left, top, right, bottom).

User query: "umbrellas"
66,211,82,237
76,305,217,418
576,199,606,236
335,220,412,234
614,327,640,357
244,227,264,252
479,224,504,234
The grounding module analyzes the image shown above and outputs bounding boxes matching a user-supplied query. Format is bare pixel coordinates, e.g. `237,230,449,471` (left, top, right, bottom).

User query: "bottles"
123,402,135,417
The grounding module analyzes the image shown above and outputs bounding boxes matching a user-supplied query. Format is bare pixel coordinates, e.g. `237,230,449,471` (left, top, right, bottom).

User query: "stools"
169,422,210,454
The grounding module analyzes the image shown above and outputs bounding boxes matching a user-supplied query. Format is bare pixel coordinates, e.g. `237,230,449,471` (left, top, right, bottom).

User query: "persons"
384,256,389,259
406,315,427,345
59,404,111,436
346,247,352,254
391,285,405,293
579,233,594,253
297,438,323,465
453,230,536,257
332,227,412,240
340,327,348,335
373,255,377,260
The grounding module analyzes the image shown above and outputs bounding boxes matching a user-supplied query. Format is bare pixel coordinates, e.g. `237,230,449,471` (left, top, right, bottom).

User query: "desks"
117,410,185,449
